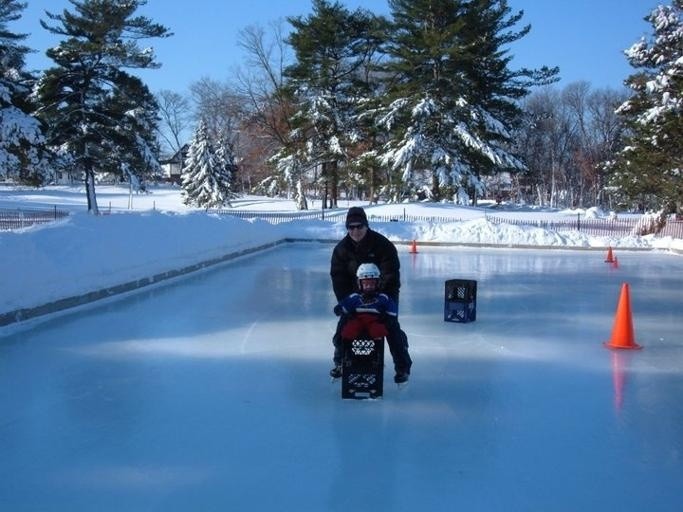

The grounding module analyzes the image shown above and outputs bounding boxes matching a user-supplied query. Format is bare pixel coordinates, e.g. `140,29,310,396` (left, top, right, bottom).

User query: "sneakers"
330,364,343,378
394,367,410,382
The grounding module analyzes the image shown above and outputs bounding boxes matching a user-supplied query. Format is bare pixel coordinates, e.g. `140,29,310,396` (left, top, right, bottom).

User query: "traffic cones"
604,247,614,263
613,256,618,268
409,240,421,254
410,253,419,270
602,344,636,413
606,262,614,273
604,282,643,353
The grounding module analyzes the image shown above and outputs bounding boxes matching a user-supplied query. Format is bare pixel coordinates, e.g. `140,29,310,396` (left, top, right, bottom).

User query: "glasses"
347,223,364,230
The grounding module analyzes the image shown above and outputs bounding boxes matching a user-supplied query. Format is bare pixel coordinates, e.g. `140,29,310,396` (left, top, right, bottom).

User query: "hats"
346,208,368,228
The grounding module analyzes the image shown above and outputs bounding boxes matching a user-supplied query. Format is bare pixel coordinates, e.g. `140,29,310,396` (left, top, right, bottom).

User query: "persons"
328,207,413,384
329,262,396,347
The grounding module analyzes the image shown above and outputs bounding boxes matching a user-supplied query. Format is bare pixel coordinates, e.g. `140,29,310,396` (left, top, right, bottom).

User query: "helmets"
356,262,383,294
